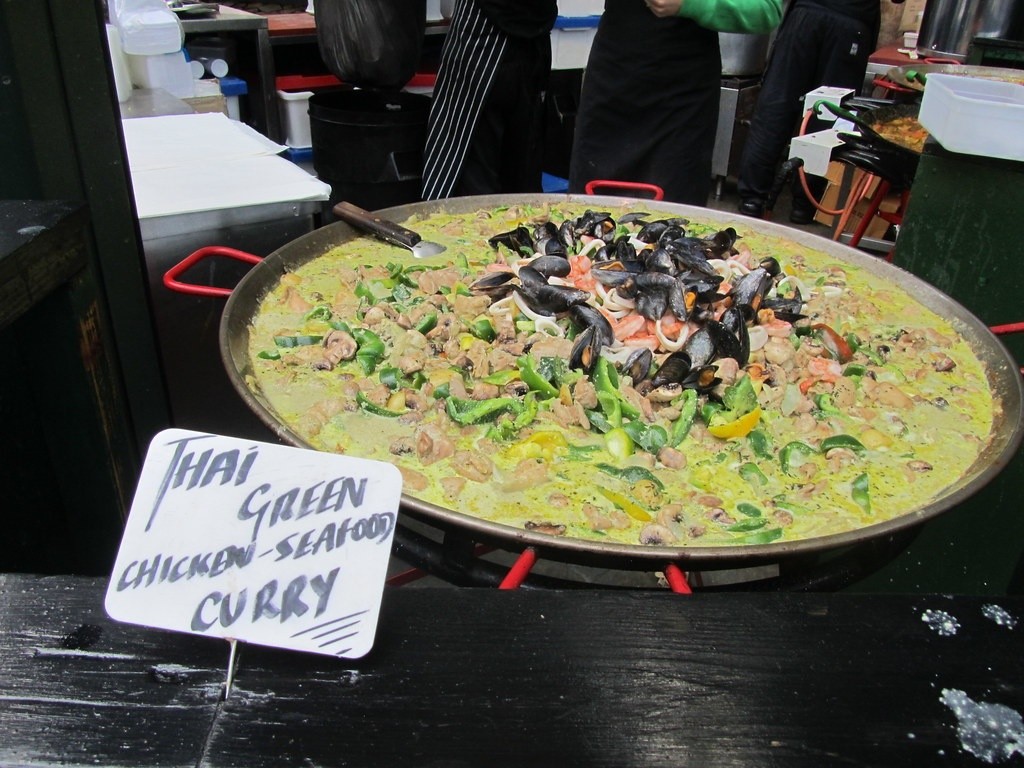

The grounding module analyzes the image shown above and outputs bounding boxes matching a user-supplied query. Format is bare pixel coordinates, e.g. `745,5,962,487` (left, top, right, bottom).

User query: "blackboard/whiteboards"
104,428,403,661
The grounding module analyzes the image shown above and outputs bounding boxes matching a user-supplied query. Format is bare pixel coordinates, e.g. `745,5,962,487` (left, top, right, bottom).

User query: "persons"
421,0,557,197
741,0,903,223
571,1,784,207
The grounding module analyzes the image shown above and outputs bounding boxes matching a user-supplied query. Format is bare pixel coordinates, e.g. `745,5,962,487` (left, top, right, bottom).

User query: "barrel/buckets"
915,1,1024,64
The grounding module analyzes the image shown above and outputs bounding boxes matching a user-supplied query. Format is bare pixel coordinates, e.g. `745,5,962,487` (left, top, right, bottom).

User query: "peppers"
261,206,900,543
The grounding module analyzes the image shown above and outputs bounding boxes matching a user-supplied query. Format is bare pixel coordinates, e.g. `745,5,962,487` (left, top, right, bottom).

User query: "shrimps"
564,254,611,296
603,306,702,354
807,356,840,377
710,281,735,322
763,319,792,337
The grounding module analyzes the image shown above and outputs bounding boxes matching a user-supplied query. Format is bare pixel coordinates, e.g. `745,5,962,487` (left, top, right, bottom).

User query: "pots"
861,103,930,155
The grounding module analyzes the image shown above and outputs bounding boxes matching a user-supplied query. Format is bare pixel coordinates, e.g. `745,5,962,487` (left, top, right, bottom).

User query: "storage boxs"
277,88,315,148
290,148,318,179
917,72,1024,162
218,75,248,122
549,16,601,71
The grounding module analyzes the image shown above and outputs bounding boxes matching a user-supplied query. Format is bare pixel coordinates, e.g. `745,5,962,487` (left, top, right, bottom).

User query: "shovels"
333,200,446,259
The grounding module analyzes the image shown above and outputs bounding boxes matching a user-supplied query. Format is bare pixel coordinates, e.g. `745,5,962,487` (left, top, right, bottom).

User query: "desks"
0,573,1023,768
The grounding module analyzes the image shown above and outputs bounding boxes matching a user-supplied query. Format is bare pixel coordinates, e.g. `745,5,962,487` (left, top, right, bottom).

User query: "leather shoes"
788,198,827,226
737,191,777,217
